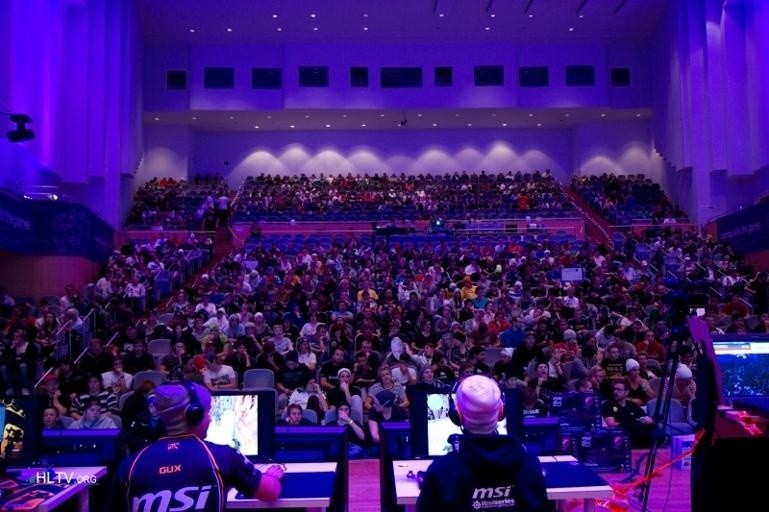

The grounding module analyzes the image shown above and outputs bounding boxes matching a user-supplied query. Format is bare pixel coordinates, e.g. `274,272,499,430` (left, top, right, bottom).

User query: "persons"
412,374,548,512
125,168,682,238
1,221,769,462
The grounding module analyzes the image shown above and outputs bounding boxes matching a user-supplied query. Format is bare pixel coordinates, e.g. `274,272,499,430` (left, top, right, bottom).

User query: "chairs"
1,174,765,448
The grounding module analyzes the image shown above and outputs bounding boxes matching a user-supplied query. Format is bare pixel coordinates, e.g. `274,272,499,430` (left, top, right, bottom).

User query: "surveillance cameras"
5,126,34,144
401,120,407,125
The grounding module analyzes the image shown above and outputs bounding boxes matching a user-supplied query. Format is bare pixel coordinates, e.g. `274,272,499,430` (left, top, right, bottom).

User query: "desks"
2,455,612,511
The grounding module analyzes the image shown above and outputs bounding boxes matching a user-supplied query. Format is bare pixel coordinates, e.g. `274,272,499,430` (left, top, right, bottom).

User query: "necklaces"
108,381,288,512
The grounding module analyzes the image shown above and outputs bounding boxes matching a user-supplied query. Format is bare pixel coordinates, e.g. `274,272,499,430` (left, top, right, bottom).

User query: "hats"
420,363,432,376
336,399,350,408
674,363,692,380
217,307,263,328
500,347,514,358
337,368,351,377
625,358,640,373
374,388,395,408
144,394,157,407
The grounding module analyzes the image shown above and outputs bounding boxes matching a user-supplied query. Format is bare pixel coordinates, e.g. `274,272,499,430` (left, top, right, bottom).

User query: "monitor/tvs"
417,388,522,463
203,387,278,465
708,332,769,416
